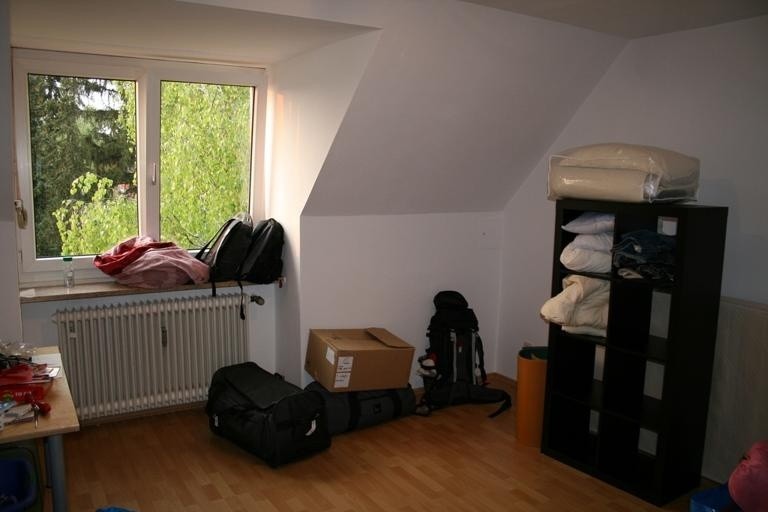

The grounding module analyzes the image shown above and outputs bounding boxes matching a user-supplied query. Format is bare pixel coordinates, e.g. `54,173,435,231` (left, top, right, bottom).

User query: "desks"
1,344,85,511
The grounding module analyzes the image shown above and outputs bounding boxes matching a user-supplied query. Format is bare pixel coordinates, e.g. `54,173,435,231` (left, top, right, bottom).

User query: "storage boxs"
303,323,416,396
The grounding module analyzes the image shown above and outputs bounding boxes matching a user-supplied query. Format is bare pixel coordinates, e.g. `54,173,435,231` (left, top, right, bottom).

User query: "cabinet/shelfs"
541,197,731,505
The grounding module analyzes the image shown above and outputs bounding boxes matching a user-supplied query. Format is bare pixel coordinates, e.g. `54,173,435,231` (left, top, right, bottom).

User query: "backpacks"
194,220,252,282
235,218,284,284
419,291,510,409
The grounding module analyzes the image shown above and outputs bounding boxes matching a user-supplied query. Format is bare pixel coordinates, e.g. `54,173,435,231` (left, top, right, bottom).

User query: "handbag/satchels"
205,362,331,466
305,379,416,436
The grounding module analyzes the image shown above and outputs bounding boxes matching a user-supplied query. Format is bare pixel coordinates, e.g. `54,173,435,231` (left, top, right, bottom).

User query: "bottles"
62,256,75,292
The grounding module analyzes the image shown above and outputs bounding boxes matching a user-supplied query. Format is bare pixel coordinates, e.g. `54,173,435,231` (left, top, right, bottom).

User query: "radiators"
53,292,264,432
